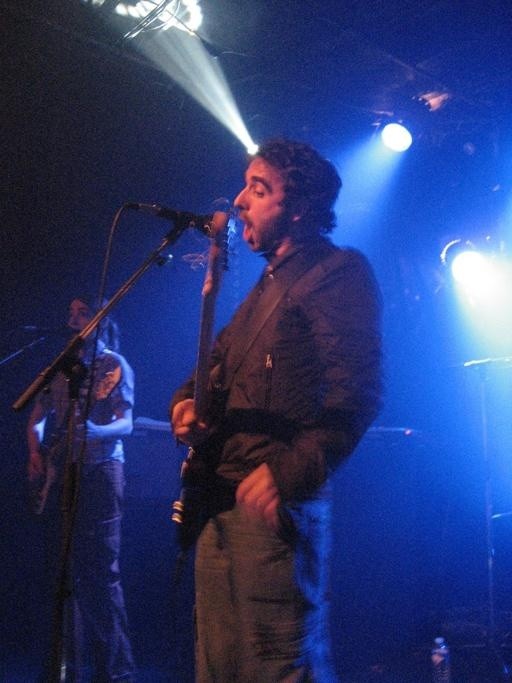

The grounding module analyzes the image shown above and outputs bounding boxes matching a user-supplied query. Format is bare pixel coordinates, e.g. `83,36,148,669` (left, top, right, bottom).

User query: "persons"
165,133,386,683
19,289,144,682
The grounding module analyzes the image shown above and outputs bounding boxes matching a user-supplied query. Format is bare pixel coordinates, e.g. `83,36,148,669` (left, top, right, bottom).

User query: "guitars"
172,212,236,550
26,365,121,515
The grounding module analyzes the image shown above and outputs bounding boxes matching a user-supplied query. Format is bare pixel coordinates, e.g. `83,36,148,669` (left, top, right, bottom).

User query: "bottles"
432,638,451,682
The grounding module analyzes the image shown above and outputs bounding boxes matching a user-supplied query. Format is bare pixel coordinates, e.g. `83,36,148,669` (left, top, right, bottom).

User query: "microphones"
156,203,214,238
20,321,74,339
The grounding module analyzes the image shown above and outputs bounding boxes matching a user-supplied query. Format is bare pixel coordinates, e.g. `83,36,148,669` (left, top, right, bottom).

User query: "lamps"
437,232,486,287
379,97,432,152
